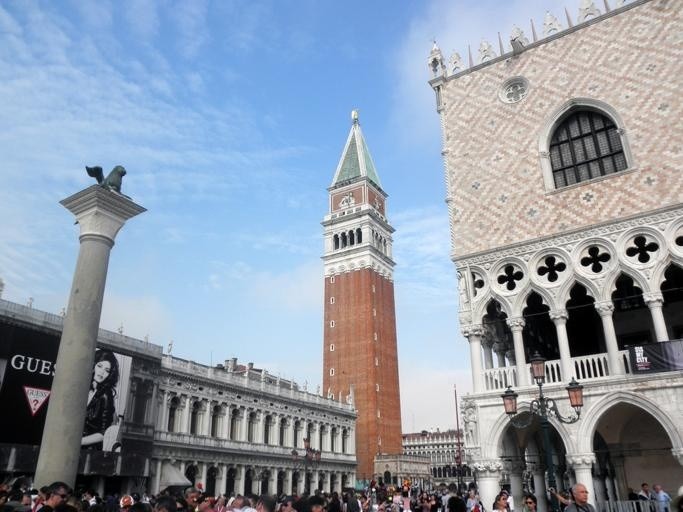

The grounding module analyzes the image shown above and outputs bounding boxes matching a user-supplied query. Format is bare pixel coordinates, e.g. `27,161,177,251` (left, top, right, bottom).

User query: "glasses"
123,504,131,509
578,490,589,494
501,497,508,501
525,501,533,504
53,491,68,498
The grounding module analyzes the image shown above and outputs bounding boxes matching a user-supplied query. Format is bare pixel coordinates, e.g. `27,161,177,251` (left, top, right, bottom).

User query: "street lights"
500,350,583,510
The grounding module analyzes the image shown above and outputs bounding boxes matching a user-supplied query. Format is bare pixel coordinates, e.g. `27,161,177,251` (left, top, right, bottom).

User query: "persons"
628,487,640,512
77,349,122,475
652,483,673,512
1,470,597,512
638,482,657,512
